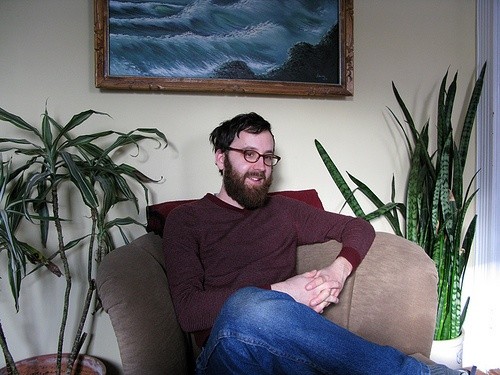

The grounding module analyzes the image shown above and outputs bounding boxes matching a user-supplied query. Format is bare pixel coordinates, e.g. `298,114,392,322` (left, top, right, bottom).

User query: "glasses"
228,147,281,166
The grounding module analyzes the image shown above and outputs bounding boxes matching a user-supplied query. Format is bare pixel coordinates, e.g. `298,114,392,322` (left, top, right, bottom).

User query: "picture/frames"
93,0,355,97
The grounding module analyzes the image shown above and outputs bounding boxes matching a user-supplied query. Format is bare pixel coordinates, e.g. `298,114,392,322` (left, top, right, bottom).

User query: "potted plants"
0,99,169,375
312,59,489,370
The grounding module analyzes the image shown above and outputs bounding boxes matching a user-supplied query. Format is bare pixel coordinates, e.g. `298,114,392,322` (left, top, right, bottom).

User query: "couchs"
95,189,439,375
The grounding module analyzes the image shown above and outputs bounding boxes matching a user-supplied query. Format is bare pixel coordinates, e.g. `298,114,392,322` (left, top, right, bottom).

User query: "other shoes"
459,365,500,375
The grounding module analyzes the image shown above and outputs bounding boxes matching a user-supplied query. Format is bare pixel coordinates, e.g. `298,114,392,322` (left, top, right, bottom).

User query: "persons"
162,112,500,375
